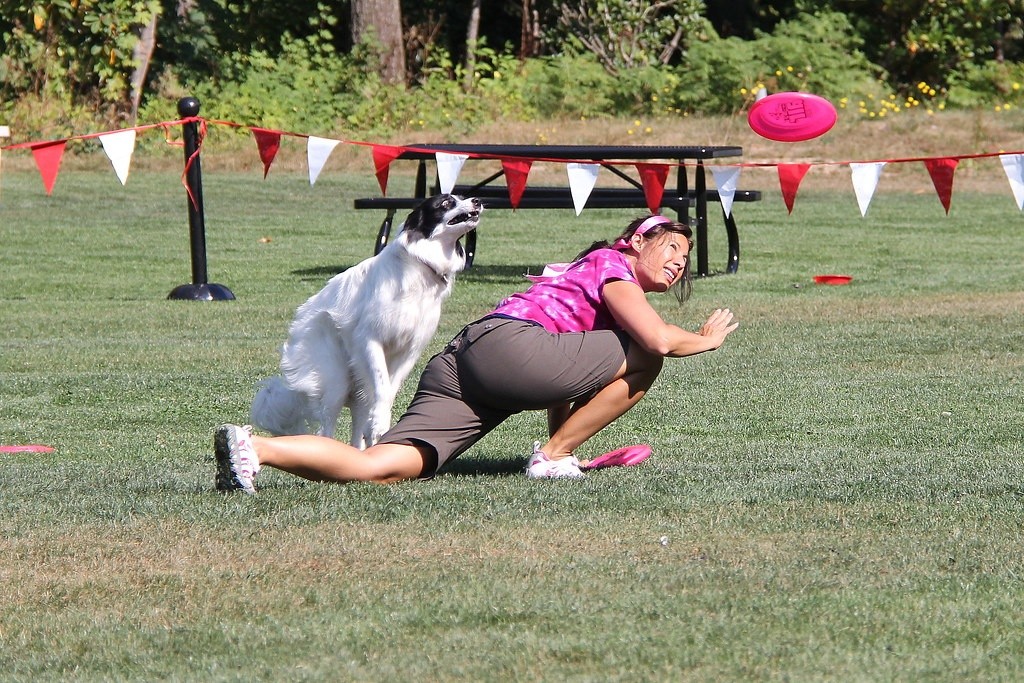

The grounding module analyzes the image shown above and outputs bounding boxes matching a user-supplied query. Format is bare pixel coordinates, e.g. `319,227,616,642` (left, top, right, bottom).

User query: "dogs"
249,193,485,449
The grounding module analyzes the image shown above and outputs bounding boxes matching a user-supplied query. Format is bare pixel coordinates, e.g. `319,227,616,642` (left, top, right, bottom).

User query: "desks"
387,144,744,277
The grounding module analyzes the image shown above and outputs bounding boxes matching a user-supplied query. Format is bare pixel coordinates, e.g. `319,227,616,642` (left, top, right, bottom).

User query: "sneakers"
527,440,585,477
213,423,261,495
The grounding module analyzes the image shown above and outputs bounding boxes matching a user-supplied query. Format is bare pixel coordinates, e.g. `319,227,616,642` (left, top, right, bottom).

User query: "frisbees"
746,91,838,144
587,445,651,469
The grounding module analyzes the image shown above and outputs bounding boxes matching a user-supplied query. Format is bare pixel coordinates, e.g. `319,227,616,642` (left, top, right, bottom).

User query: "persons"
215,214,740,490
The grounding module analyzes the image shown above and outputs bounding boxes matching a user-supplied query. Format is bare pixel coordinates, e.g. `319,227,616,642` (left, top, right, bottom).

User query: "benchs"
355,186,762,281
